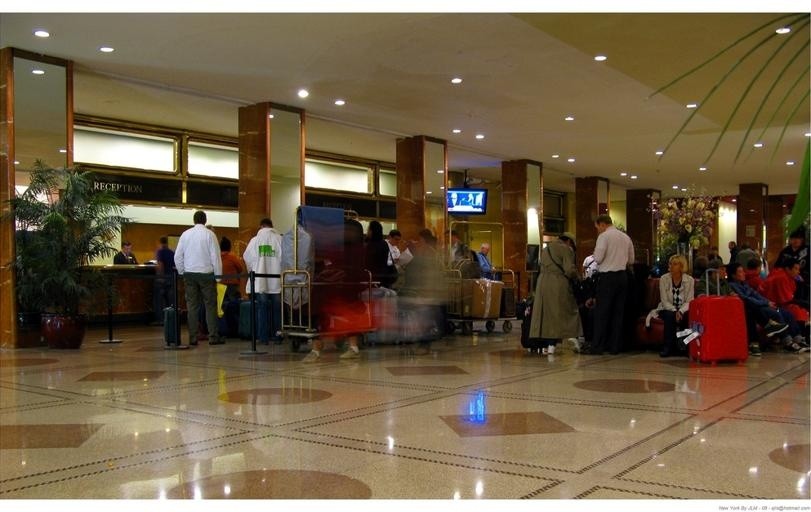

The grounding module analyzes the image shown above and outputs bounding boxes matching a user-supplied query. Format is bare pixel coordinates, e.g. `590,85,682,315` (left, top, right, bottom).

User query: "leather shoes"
209,340,226,345
660,348,672,357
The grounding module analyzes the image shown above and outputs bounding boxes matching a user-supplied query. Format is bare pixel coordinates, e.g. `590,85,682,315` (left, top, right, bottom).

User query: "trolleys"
279,205,375,347
448,218,516,335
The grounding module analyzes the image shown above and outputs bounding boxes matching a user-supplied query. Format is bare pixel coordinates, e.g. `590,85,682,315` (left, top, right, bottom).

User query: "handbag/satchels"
450,258,480,278
573,277,592,305
385,251,398,286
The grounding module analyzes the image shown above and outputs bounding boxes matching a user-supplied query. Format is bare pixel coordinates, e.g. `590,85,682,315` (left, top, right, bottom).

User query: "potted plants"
5,188,54,348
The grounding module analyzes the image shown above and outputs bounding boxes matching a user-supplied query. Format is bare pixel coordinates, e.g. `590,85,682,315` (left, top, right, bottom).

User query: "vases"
676,241,694,277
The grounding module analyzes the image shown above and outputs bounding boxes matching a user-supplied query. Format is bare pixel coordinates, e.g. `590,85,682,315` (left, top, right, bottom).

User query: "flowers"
645,182,727,255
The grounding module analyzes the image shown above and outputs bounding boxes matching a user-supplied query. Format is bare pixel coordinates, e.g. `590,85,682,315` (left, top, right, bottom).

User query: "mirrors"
760,183,767,257
0,156,136,350
423,134,447,266
526,159,543,274
267,101,305,238
597,176,610,215
7,47,73,251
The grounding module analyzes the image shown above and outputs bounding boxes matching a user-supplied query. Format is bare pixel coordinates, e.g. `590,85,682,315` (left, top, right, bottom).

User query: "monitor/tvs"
447,188,487,214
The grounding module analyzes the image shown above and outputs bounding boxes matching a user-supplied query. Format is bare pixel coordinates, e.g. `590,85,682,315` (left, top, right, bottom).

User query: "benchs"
638,277,705,353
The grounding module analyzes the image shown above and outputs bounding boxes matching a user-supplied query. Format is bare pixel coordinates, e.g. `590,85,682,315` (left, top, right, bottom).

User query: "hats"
560,232,577,250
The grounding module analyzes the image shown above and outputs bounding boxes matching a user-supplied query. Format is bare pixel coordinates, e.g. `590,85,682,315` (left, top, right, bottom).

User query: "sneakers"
340,347,360,359
301,350,320,363
567,338,581,352
796,342,810,351
765,324,788,338
783,342,806,354
748,345,761,356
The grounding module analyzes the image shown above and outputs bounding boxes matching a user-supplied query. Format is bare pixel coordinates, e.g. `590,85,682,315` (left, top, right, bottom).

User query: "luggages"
521,297,540,351
501,287,514,317
218,297,240,337
239,300,259,341
164,307,182,343
687,268,747,366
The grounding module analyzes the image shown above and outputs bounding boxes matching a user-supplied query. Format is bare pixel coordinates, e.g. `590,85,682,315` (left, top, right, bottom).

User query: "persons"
243,218,283,346
446,230,469,278
216,237,243,340
657,255,694,358
448,192,457,208
173,211,226,346
587,214,635,355
528,232,582,355
364,221,389,286
149,236,177,326
113,240,138,265
384,230,400,272
419,230,436,268
301,204,364,363
476,244,496,281
692,233,811,356
